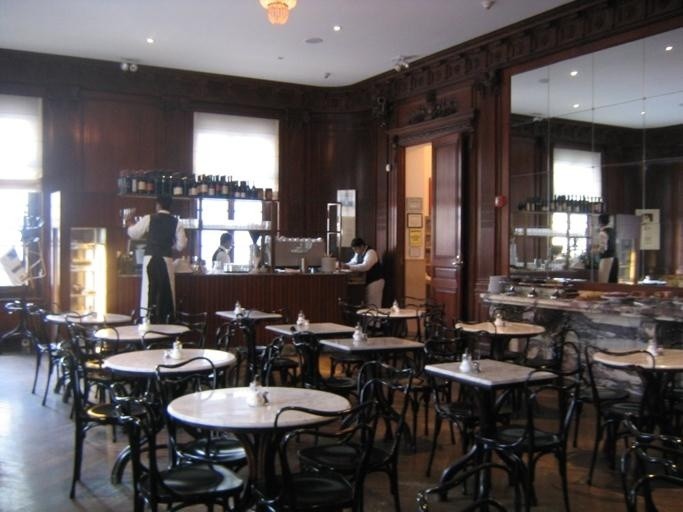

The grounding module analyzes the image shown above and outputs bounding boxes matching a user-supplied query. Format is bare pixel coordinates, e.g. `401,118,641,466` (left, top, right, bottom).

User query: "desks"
116,269,359,348
480,293,682,322
478,280,683,358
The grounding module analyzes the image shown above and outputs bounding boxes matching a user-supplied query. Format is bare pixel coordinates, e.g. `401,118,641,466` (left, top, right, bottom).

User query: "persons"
211,232,234,271
126,192,188,325
589,213,620,284
339,236,385,310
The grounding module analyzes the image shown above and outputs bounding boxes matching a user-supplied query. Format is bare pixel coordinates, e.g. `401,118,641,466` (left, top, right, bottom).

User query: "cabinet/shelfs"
522,210,601,268
118,192,280,267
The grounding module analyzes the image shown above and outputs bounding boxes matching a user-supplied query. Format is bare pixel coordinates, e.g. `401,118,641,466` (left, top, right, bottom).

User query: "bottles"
247,375,265,407
353,321,367,342
234,299,243,315
143,314,150,330
459,348,472,373
220,176,228,197
250,186,258,199
119,169,215,197
494,314,502,326
240,181,247,199
215,176,221,196
234,181,239,198
227,175,234,197
246,181,250,198
172,336,184,359
390,297,400,313
297,310,305,331
646,338,657,357
518,195,605,213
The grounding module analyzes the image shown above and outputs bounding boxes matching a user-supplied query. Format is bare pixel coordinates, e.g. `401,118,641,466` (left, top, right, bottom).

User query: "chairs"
215,322,243,357
622,443,683,511
423,334,514,495
399,295,437,313
71,366,151,512
492,307,536,324
22,301,58,394
140,330,204,348
156,358,248,472
416,304,446,340
342,302,378,331
476,364,587,511
131,304,157,324
39,311,81,406
584,346,655,485
166,310,208,334
242,318,299,386
251,401,381,512
624,410,681,445
293,331,357,392
110,382,244,512
298,361,415,512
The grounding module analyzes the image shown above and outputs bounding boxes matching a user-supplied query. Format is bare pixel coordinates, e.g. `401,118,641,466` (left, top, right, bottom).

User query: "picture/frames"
408,212,423,228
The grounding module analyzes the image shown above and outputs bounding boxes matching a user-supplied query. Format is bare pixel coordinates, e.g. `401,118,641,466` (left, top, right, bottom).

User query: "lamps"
260,0,297,25
